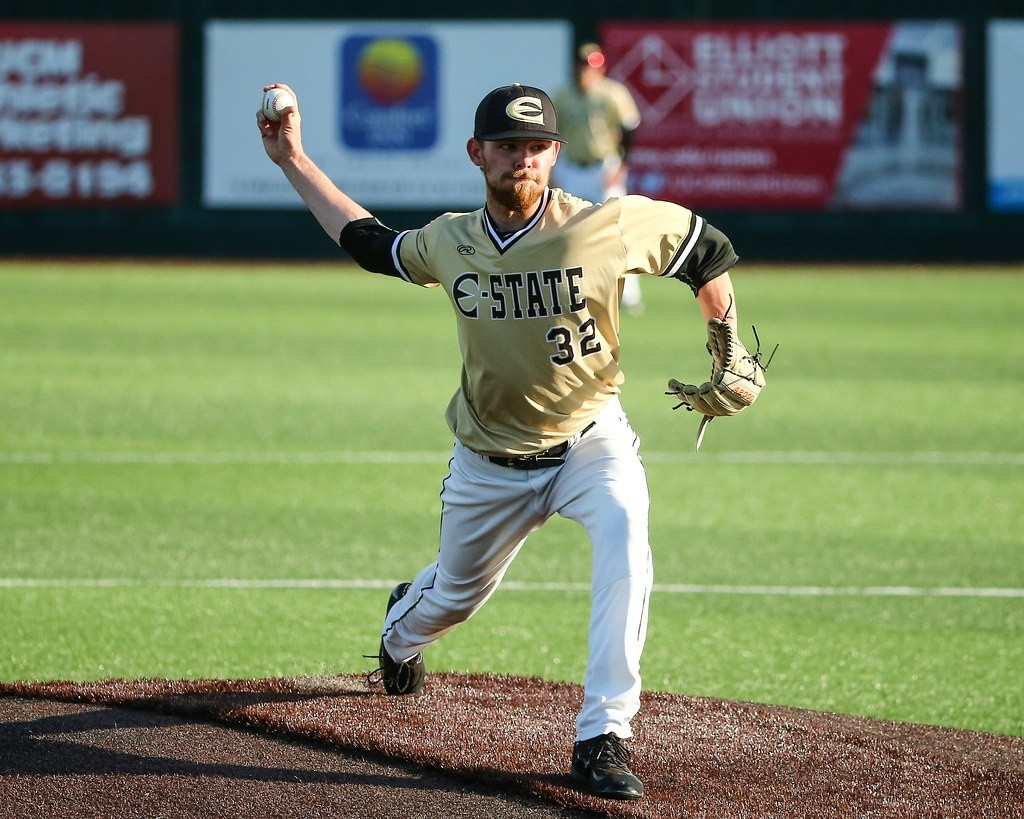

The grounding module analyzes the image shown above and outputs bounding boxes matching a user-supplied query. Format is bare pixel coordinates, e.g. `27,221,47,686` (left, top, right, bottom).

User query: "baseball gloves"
666,315,767,418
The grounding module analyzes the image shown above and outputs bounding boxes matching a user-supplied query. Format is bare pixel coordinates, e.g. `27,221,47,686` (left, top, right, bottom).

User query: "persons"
549,43,644,317
256,83,767,799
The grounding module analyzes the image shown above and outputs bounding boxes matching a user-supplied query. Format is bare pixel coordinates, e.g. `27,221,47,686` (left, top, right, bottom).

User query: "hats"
474,84,568,145
577,43,603,63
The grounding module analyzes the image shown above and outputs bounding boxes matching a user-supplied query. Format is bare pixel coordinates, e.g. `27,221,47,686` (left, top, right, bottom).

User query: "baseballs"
261,89,295,122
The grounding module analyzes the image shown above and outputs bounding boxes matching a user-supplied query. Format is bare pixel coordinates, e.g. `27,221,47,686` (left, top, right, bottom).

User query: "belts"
464,422,594,471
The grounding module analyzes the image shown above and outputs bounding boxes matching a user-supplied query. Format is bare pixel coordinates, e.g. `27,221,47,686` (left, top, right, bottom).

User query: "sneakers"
566,731,644,801
362,584,425,696
